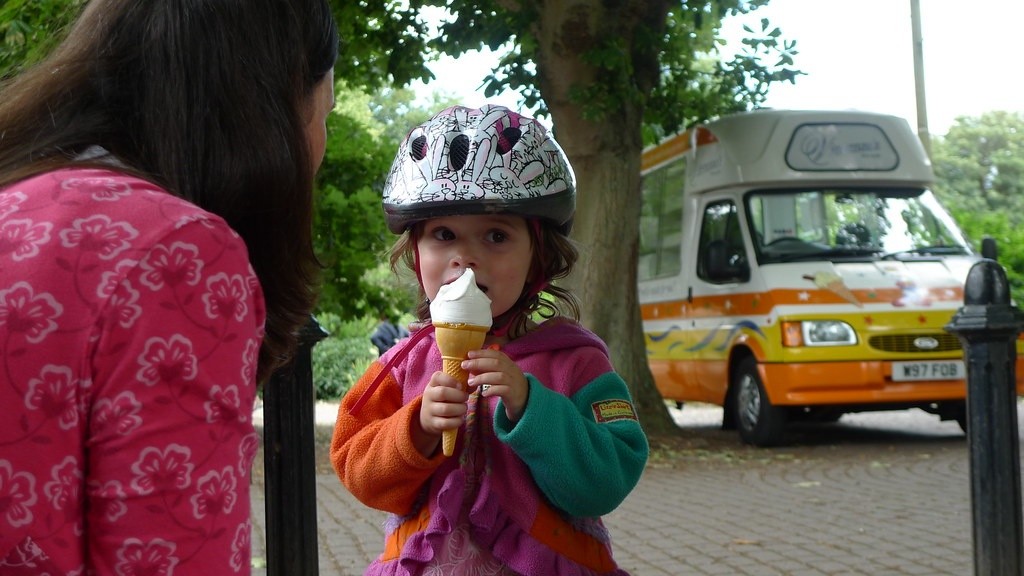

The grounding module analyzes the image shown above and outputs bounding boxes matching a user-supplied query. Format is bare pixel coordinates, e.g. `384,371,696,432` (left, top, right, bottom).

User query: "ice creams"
811,268,863,307
427,268,492,455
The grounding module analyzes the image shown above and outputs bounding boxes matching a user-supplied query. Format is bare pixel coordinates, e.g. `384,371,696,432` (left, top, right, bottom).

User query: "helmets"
382,105,577,246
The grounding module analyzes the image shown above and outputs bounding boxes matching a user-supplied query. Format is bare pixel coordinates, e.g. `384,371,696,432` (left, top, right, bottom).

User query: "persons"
372,311,408,356
0,0,338,574
327,104,650,575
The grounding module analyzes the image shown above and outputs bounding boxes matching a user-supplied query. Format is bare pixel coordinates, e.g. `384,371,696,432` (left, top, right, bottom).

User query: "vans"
632,109,1018,445
